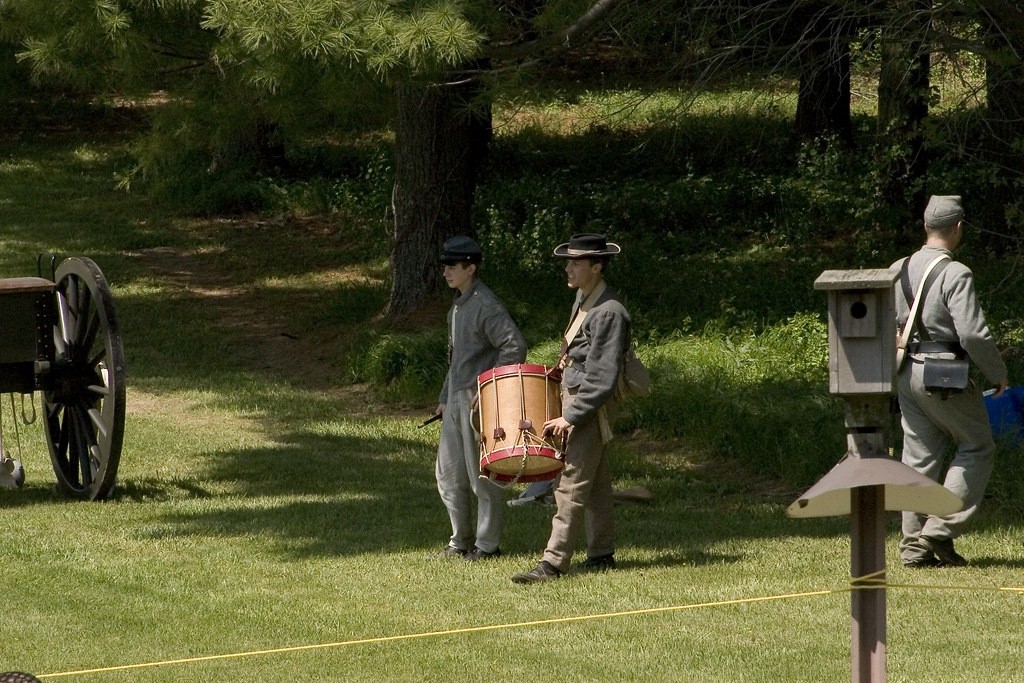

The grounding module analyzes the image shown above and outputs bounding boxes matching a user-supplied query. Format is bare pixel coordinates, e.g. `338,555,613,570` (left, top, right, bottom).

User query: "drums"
476,363,566,484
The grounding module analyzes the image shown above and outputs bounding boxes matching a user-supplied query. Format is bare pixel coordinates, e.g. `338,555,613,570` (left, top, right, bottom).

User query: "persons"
887,195,1007,569
510,233,633,583
434,235,527,562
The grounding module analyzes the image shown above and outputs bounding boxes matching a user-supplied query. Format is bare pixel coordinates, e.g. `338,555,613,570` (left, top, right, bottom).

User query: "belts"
906,340,968,360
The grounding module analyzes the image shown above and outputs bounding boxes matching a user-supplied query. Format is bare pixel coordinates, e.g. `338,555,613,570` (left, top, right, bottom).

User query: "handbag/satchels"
623,357,651,397
896,335,908,374
612,369,631,404
923,358,970,392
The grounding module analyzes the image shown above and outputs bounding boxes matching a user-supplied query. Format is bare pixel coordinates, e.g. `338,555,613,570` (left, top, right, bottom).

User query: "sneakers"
576,553,617,570
463,546,502,560
511,560,565,584
439,547,468,557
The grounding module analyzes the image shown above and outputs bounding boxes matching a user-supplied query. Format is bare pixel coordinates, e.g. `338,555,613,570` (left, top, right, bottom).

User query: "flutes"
418,414,441,429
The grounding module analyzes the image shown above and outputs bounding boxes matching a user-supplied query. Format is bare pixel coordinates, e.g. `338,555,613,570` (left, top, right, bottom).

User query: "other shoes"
918,535,967,566
903,556,942,567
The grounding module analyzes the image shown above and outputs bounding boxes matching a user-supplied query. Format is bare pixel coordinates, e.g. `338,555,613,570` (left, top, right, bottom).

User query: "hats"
553,233,621,257
924,195,965,228
439,236,482,266
